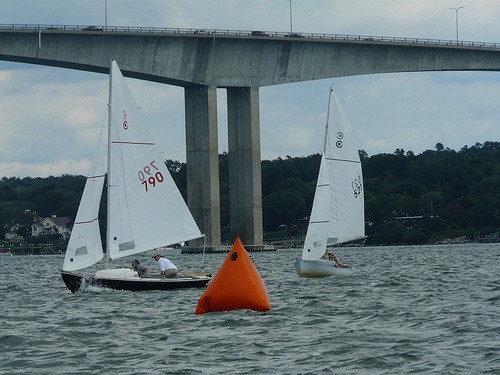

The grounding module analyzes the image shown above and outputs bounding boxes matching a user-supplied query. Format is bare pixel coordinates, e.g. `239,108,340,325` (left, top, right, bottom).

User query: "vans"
44,27,61,29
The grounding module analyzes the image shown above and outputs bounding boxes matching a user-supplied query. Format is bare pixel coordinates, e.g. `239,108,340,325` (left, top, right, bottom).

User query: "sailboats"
57,59,213,294
293,82,368,278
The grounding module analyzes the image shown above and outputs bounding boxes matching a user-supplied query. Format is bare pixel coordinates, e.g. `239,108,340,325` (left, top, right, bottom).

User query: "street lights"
449,6,465,45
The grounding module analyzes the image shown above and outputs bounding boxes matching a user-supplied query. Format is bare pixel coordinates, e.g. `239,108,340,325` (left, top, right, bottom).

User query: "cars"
284,33,305,38
81,26,103,31
362,37,377,41
193,29,211,35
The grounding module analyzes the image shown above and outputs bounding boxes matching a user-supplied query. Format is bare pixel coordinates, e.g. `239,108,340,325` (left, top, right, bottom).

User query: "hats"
152,253,160,258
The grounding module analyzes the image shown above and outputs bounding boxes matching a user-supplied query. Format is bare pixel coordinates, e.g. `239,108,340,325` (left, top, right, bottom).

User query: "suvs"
251,31,270,36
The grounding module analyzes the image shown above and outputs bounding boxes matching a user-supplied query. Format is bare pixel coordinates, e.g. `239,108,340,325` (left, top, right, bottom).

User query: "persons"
132,259,150,277
324,249,339,264
152,253,178,277
